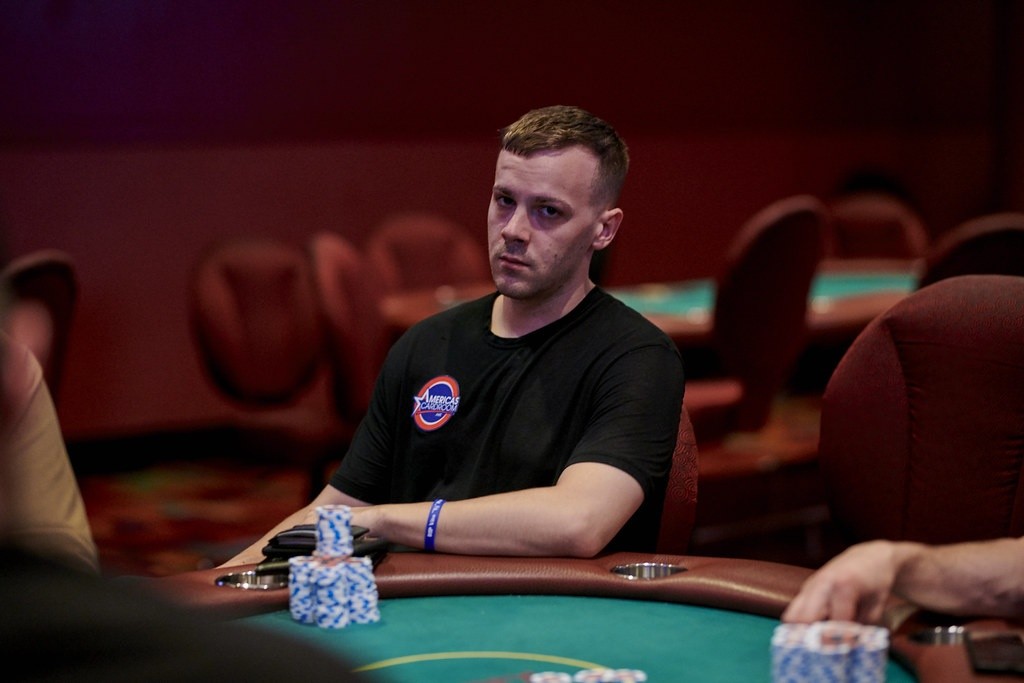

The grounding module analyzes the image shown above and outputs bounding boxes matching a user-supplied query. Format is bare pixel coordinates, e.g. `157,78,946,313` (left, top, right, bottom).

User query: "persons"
0,329,374,683
211,106,684,570
781,535,1024,645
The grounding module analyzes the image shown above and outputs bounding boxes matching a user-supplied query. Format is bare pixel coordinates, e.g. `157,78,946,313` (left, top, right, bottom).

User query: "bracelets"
424,499,446,552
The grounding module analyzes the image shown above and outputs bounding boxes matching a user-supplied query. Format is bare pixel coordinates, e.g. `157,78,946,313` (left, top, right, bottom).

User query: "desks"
160,551,1024,683
374,251,930,386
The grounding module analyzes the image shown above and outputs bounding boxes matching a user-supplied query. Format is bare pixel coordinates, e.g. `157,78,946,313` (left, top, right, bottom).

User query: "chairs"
192,187,1024,631
